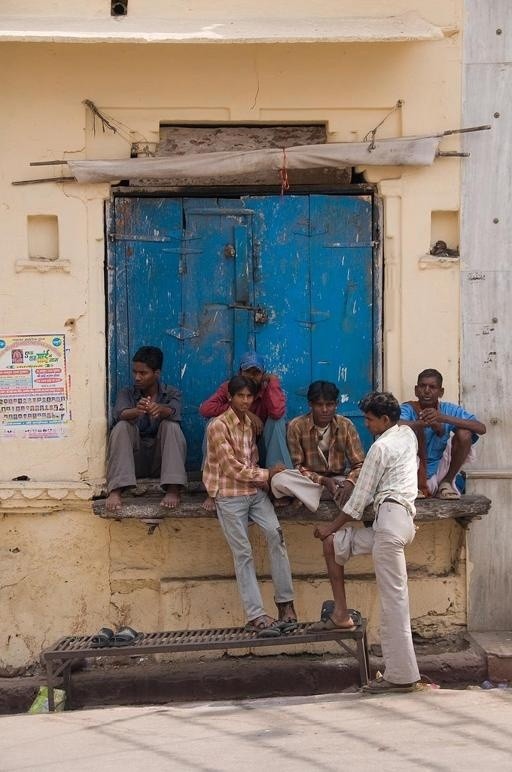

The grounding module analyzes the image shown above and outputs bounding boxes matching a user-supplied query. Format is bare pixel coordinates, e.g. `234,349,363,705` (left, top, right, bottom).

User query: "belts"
383,498,396,503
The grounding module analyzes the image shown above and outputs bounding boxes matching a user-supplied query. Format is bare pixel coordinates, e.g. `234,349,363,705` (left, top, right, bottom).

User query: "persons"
202,374,298,633
270,380,366,513
396,369,486,501
313,391,421,695
200,353,294,510
106,346,187,513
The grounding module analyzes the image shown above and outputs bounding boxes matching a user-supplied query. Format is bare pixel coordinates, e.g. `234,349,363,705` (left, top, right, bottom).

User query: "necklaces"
314,424,331,440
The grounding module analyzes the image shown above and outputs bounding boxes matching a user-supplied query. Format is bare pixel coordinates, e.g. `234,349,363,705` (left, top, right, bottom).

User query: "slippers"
277,621,302,633
88,627,112,647
327,612,329,613
275,603,298,630
417,489,426,499
256,629,280,638
304,616,357,634
244,614,280,632
108,627,143,647
320,598,335,621
361,678,416,695
347,607,362,626
435,482,461,500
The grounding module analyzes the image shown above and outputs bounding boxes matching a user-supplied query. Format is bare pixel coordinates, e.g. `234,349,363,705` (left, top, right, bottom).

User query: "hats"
240,351,265,372
